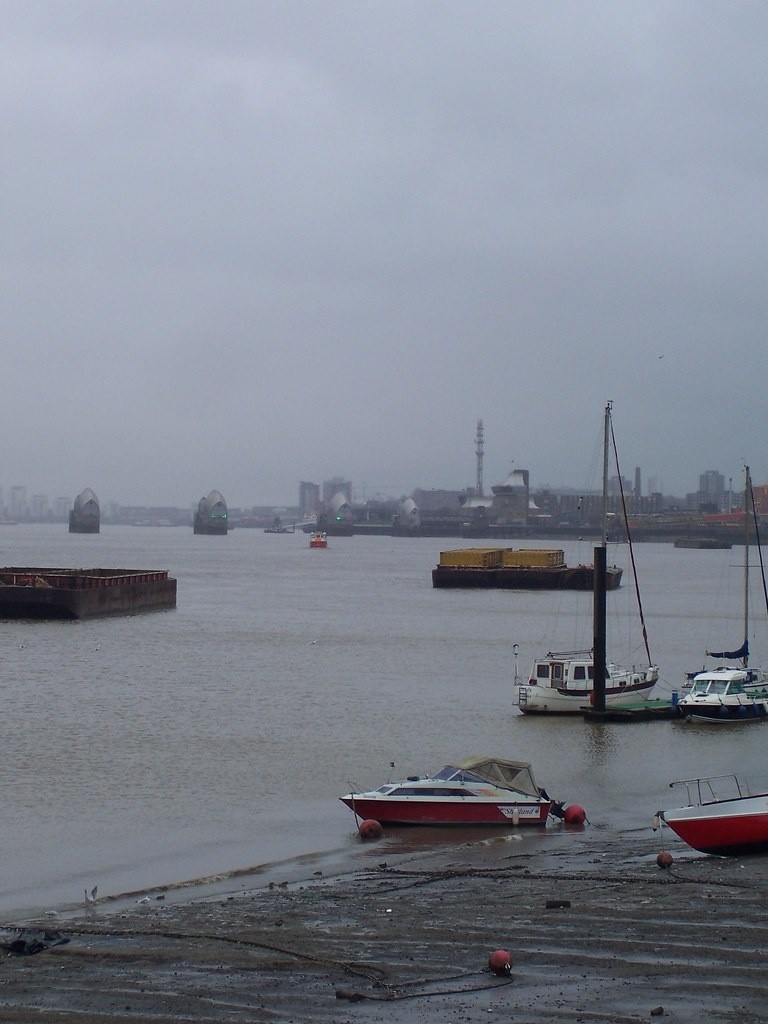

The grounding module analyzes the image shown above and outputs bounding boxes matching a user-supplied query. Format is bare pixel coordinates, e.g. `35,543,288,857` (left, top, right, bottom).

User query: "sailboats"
673,459,768,726
510,398,662,721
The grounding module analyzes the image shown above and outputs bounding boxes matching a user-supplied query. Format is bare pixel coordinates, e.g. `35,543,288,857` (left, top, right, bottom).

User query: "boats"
192,488,229,536
429,547,625,592
389,498,422,536
67,487,101,534
309,531,328,547
337,755,567,829
0,564,177,619
653,773,768,857
264,516,297,535
301,491,356,537
673,536,733,550
